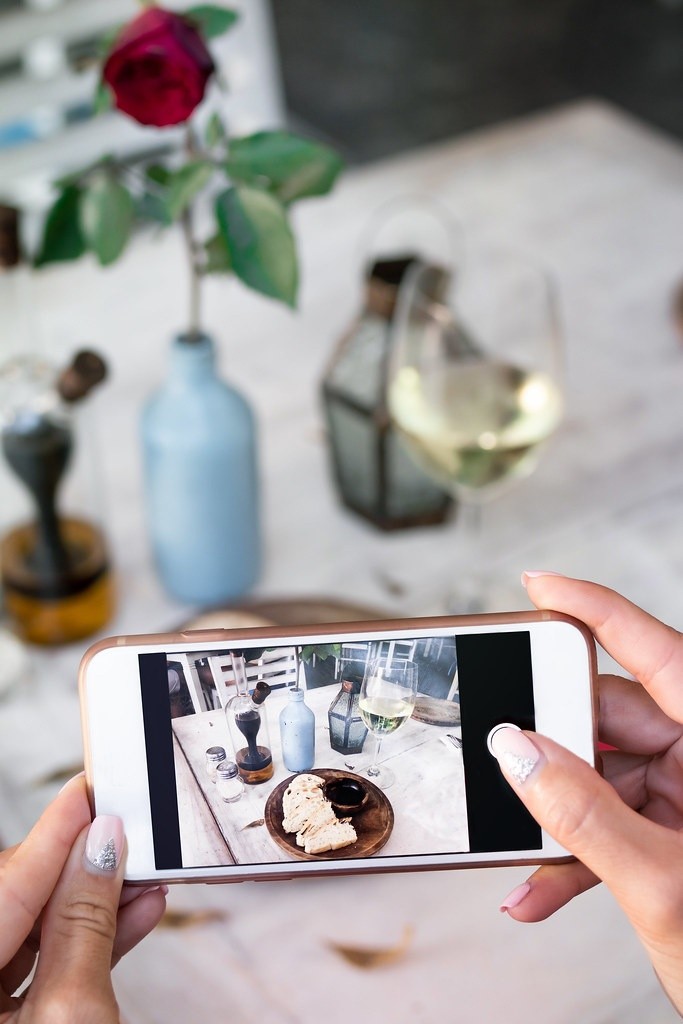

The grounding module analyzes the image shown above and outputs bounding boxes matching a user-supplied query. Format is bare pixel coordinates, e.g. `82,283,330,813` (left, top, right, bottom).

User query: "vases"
136,337,262,603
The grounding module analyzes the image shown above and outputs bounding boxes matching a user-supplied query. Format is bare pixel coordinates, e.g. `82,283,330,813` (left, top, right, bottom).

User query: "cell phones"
78,609,604,888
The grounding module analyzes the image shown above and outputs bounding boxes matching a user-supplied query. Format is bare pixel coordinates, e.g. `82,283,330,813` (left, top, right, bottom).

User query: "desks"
167,653,217,713
0,104,683,1024
171,678,470,869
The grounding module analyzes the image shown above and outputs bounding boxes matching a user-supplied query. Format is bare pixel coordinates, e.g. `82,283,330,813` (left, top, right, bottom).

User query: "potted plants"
232,645,343,774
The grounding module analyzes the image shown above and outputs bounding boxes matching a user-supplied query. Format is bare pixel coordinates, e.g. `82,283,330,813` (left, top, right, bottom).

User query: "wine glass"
358,658,418,789
383,251,560,623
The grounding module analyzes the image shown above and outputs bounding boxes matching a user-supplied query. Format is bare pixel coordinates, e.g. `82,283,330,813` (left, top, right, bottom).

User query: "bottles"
205,746,229,785
216,761,245,803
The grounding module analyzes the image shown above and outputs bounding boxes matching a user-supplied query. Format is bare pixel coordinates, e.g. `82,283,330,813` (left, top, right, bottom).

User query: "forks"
449,736,462,748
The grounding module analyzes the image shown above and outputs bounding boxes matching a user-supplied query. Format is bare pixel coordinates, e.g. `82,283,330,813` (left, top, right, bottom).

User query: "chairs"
212,655,292,711
376,640,418,678
446,669,459,704
312,653,339,680
431,638,456,660
206,647,307,712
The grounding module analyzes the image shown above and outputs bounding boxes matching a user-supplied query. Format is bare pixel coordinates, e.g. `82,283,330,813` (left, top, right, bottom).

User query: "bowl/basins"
324,777,370,816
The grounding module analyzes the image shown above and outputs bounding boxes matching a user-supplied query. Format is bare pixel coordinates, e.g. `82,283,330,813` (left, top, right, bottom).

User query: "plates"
264,768,394,861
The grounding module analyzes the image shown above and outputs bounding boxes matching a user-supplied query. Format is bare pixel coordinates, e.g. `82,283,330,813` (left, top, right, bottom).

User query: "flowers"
34,8,337,340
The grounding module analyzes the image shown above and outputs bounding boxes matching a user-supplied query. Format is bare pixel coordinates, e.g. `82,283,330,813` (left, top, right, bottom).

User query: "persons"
0,570,683,1023
